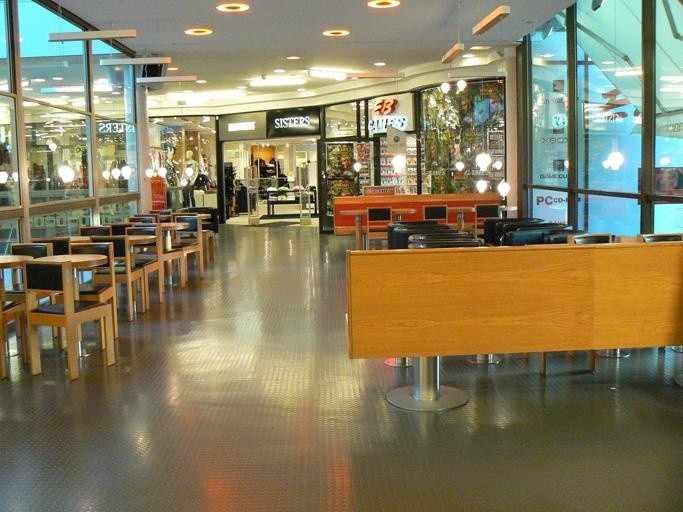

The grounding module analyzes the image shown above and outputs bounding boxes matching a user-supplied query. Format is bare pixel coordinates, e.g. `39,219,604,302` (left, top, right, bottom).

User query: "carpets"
260,214,320,219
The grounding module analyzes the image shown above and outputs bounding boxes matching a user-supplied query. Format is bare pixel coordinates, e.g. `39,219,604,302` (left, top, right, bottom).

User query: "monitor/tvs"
139,53,168,89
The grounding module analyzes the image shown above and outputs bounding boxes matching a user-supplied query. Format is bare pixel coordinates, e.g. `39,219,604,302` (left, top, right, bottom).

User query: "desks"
340,209,369,250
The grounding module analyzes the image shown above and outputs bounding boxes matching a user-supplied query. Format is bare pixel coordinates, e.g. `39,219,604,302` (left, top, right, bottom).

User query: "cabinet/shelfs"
357,138,424,194
267,190,317,217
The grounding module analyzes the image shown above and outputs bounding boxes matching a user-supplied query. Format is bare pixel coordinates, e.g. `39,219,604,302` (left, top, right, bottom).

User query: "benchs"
343,241,682,412
333,192,502,236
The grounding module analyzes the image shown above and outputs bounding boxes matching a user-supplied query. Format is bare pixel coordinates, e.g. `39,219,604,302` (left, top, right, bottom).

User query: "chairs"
366,204,683,377
0,206,220,382
